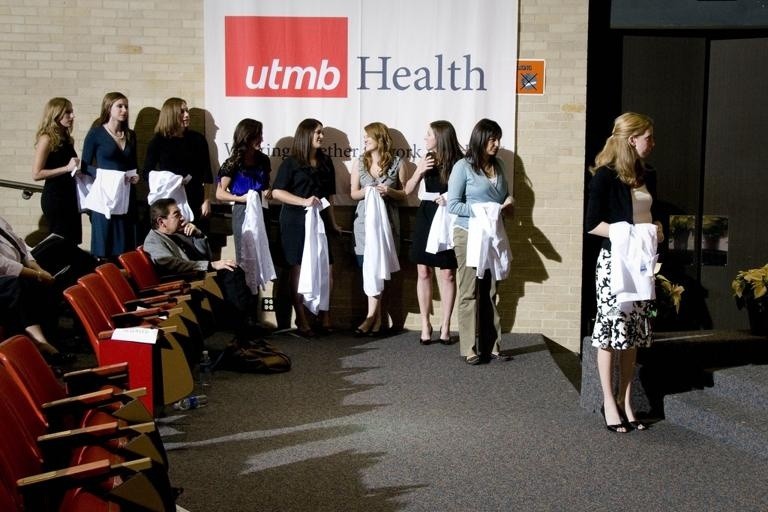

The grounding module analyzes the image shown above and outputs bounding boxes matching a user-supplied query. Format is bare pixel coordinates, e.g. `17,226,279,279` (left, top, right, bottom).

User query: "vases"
746,303,768,337
673,234,688,249
705,234,720,250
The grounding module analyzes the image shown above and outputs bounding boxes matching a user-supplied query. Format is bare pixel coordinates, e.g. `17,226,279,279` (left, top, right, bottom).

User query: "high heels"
420,326,433,345
615,400,646,432
367,327,382,338
354,327,365,336
295,319,314,337
440,326,451,345
601,402,629,433
317,319,334,334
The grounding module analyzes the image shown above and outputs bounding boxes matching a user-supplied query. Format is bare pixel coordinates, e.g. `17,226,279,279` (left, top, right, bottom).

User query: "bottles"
197,350,211,386
172,397,208,412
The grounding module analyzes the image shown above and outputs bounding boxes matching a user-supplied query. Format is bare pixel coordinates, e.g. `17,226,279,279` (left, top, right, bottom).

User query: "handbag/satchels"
236,338,292,375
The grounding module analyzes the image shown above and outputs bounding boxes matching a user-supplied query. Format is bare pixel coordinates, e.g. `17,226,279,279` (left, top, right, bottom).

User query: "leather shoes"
487,351,510,361
464,355,480,365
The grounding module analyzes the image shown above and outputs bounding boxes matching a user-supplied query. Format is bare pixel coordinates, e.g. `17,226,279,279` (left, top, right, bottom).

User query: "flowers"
657,275,686,314
669,215,729,237
731,257,768,302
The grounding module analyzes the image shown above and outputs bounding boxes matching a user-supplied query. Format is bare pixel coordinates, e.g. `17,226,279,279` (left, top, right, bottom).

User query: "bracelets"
38,271,42,283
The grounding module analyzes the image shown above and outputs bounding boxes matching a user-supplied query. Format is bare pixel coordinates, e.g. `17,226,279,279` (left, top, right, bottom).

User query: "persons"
31,94,82,247
270,119,343,337
142,96,213,227
213,116,278,320
0,215,72,339
0,274,78,366
584,107,663,436
142,197,270,346
347,114,408,338
446,118,515,365
79,92,139,258
404,119,469,344
